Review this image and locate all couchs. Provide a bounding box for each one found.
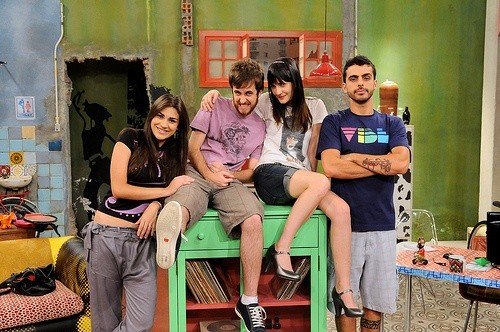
[0,236,93,332]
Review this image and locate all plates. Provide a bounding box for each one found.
[24,213,57,224]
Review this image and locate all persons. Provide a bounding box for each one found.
[79,93,195,332]
[152,56,267,332]
[200,56,366,319]
[314,55,410,332]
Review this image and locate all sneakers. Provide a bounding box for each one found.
[156,201,188,269]
[235,297,267,332]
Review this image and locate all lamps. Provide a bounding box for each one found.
[309,0,342,79]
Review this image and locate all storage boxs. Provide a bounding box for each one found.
[468,227,487,252]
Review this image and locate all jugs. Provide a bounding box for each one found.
[380,81,398,118]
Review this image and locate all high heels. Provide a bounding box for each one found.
[332,286,364,317]
[263,243,300,281]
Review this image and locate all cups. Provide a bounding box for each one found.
[449,254,465,273]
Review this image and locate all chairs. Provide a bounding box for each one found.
[459,221,500,332]
[397,209,438,314]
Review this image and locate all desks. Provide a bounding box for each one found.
[396,245,500,332]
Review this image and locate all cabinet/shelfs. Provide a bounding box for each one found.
[168,204,329,332]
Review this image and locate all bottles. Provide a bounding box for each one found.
[402,107,410,124]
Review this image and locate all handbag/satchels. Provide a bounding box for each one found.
[11,264,56,296]
